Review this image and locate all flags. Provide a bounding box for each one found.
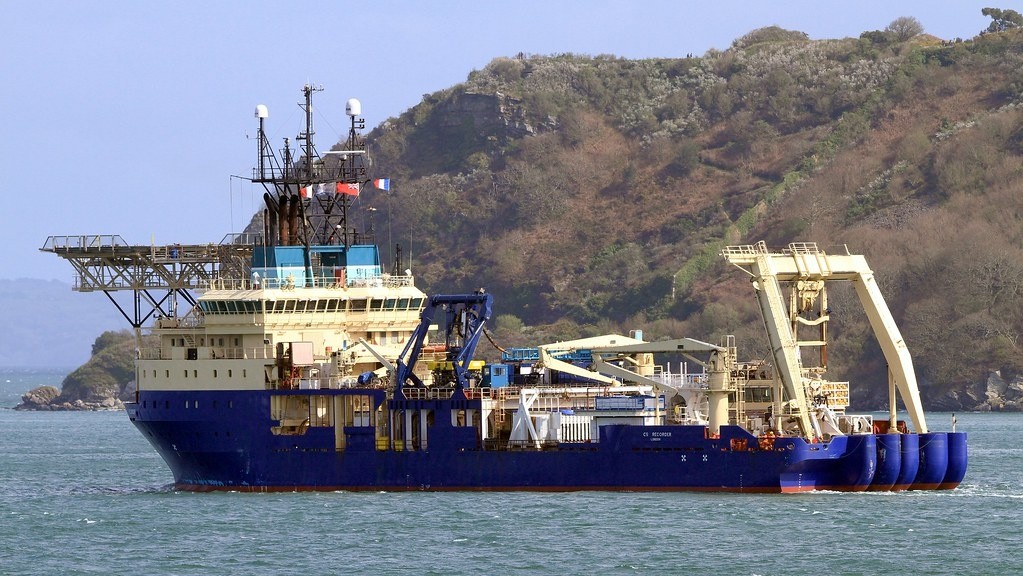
[372,177,392,192]
[336,181,360,198]
[314,181,336,200]
[299,184,317,200]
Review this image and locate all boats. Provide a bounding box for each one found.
[39,84,967,492]
